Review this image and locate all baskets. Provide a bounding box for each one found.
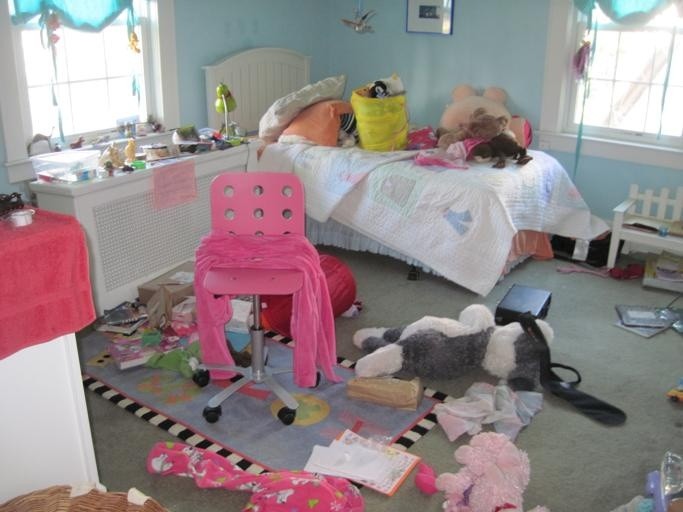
[1,485,169,512]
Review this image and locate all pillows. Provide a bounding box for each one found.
[276,98,353,147]
[256,68,347,141]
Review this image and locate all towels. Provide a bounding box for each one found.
[186,225,345,390]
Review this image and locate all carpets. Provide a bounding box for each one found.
[70,302,448,501]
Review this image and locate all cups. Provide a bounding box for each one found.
[10,208,35,227]
[658,226,670,236]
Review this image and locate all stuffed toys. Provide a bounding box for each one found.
[352,303,556,391]
[414,430,550,511]
[336,71,533,168]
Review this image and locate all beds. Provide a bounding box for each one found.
[197,40,613,297]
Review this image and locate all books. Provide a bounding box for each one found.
[613,248,683,338]
[101,322,186,371]
[92,300,149,336]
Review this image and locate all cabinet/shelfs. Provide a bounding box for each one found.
[0,205,102,508]
[24,130,251,316]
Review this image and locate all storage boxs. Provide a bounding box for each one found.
[133,259,197,309]
[217,298,253,353]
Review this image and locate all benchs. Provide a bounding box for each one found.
[600,180,681,277]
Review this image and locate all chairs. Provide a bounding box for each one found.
[187,170,321,424]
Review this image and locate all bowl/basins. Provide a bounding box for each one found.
[660,451,683,494]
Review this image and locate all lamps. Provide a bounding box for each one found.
[210,76,240,143]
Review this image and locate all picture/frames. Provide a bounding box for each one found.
[399,0,458,39]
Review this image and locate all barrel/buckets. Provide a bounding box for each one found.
[350,89,409,150]
